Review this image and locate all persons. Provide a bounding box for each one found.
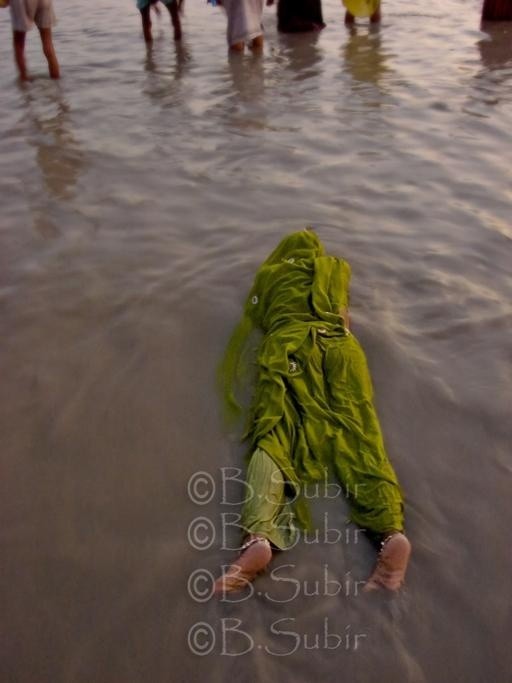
[223,57,268,131]
[341,0,381,26]
[207,0,264,55]
[474,27,512,74]
[142,50,188,110]
[135,0,187,50]
[19,81,84,200]
[0,0,60,81]
[346,25,384,85]
[211,228,411,595]
[481,0,512,26]
[266,0,327,34]
